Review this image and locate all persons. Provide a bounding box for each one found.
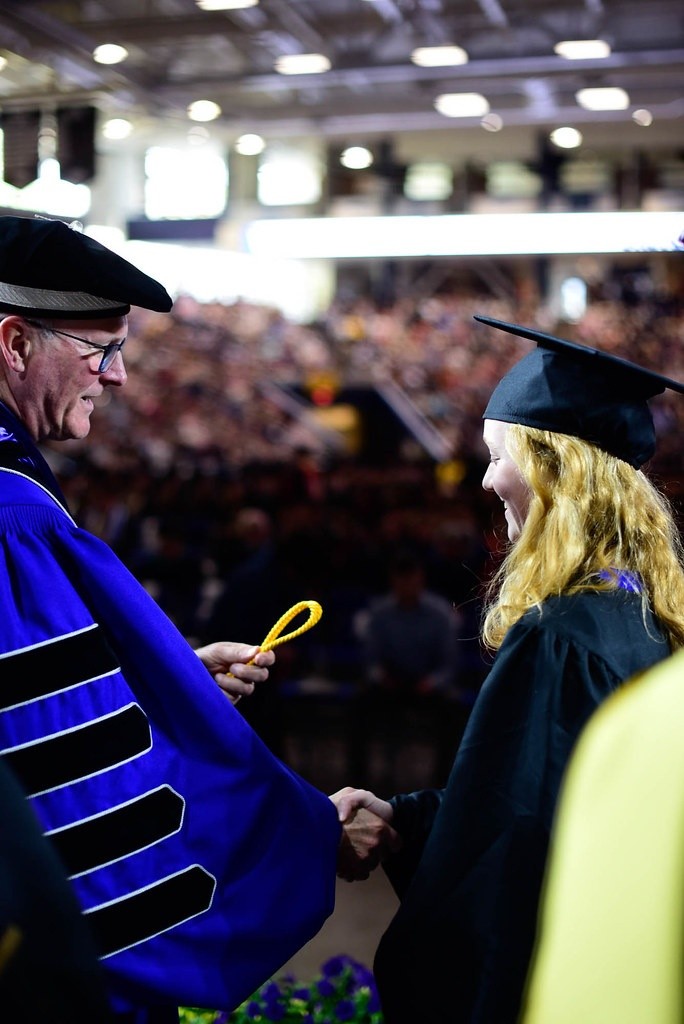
[337,350,683,1024]
[44,290,683,802]
[0,248,392,1024]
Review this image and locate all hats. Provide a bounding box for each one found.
[471,316,683,471]
[1,208,173,321]
